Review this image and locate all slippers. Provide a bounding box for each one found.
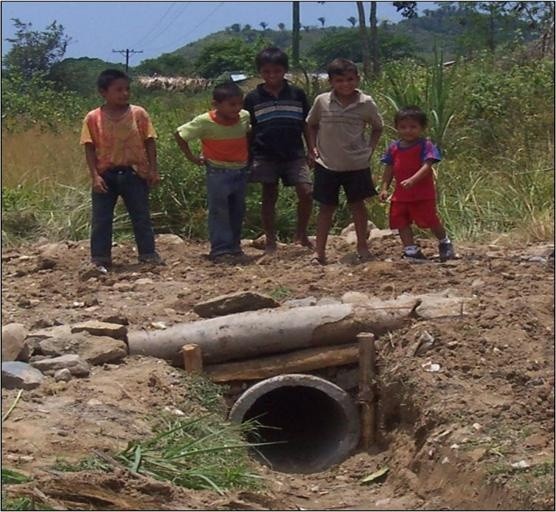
[311,254,326,266]
[296,241,314,249]
[357,251,374,262]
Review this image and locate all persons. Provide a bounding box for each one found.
[243,47,316,254]
[380,104,458,262]
[304,56,383,264]
[78,66,166,274]
[169,80,254,263]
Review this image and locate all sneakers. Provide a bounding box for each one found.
[231,253,248,265]
[137,251,164,264]
[214,253,232,264]
[438,238,454,262]
[405,244,422,258]
[91,255,111,268]
